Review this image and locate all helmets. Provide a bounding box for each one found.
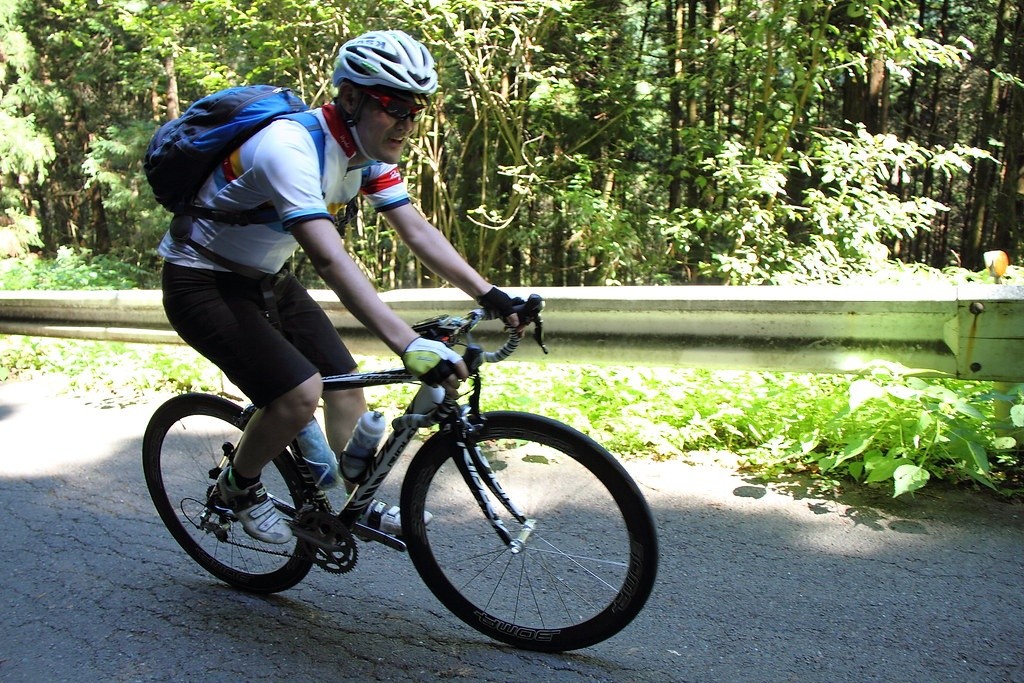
[333,29,438,110]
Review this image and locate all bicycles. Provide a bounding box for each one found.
[141,292,660,651]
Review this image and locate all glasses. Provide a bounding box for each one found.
[364,91,430,125]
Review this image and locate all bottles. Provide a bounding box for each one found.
[339,410,386,482]
[295,413,339,490]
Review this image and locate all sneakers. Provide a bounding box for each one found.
[353,498,433,542]
[218,467,294,546]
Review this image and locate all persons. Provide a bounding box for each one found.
[154,30,536,546]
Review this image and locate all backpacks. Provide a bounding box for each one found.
[145,83,323,231]
